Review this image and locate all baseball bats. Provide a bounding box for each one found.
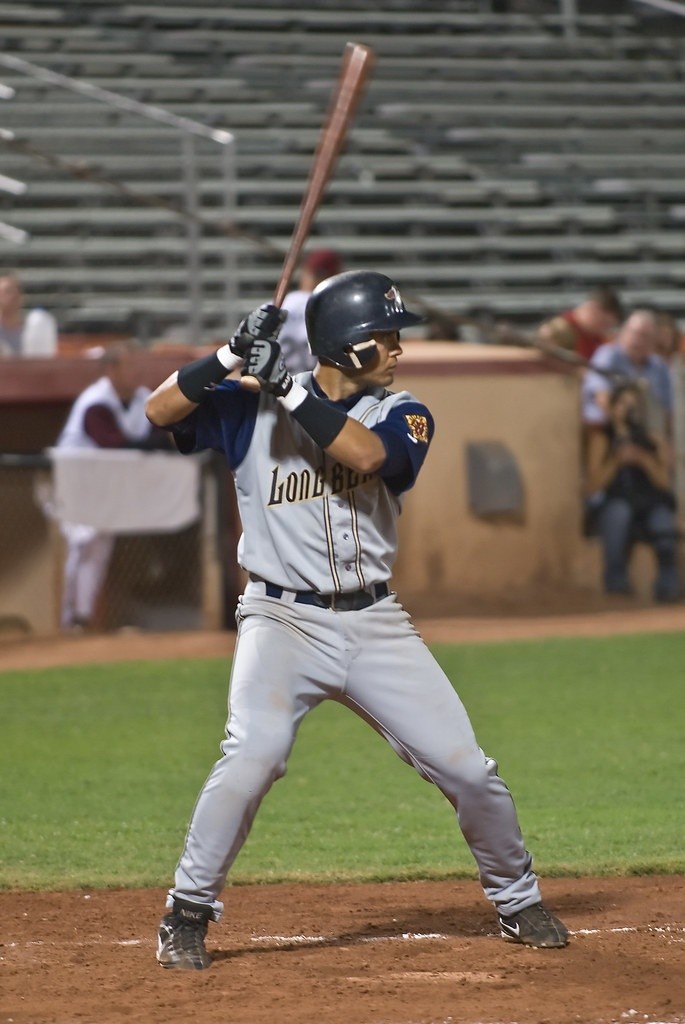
[239,40,380,392]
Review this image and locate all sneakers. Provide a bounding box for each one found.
[496,902,571,948]
[157,898,214,970]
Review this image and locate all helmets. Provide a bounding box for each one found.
[304,270,425,370]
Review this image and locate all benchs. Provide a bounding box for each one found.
[0,0,685,341]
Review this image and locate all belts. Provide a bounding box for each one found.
[263,580,389,612]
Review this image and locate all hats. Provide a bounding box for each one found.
[307,249,338,275]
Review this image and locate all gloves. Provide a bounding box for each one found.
[240,339,294,398]
[228,304,289,352]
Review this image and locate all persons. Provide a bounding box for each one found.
[52,336,177,637]
[144,269,572,969]
[0,271,66,361]
[261,250,348,380]
[536,283,684,607]
